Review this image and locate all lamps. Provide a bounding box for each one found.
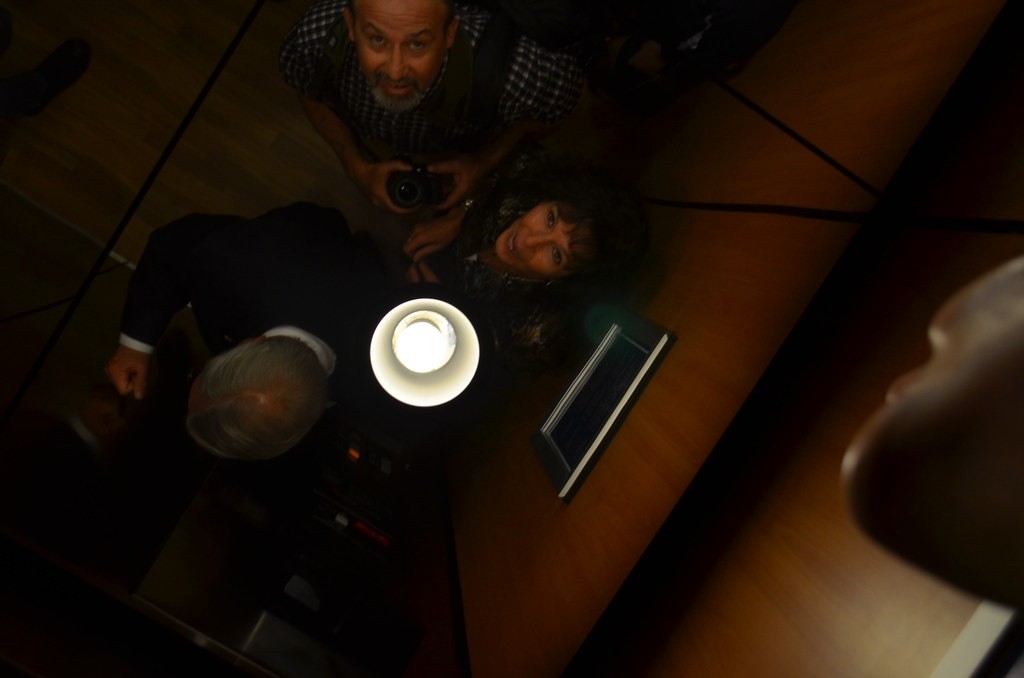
[369,297,480,409]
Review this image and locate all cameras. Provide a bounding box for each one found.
[387,163,443,209]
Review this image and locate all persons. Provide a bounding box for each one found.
[837,257,1024,617]
[106,0,794,489]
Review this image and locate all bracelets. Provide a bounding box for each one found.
[461,198,477,210]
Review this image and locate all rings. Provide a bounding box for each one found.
[408,236,412,241]
